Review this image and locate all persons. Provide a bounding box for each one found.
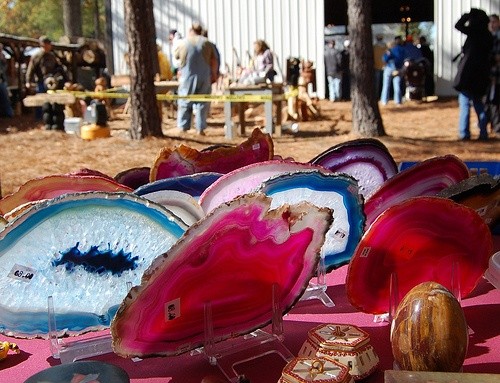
[174,25,218,136]
[487,14,500,134]
[242,40,318,119]
[451,8,495,141]
[323,32,434,106]
[0,36,117,134]
[156,29,182,122]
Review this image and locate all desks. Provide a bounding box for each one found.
[224,85,283,141]
[0,263,500,383]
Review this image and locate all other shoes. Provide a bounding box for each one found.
[458,137,470,141]
[479,135,488,140]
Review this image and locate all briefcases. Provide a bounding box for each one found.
[86,99,107,126]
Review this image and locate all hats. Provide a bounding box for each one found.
[39,36,51,43]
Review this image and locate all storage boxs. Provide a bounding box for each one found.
[277,324,378,382]
